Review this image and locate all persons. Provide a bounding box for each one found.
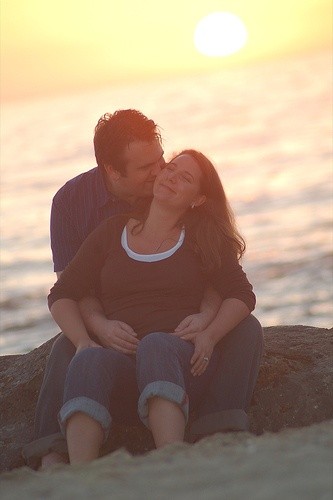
[19,109,264,474]
[46,148,258,467]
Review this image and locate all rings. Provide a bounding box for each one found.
[204,358,209,362]
